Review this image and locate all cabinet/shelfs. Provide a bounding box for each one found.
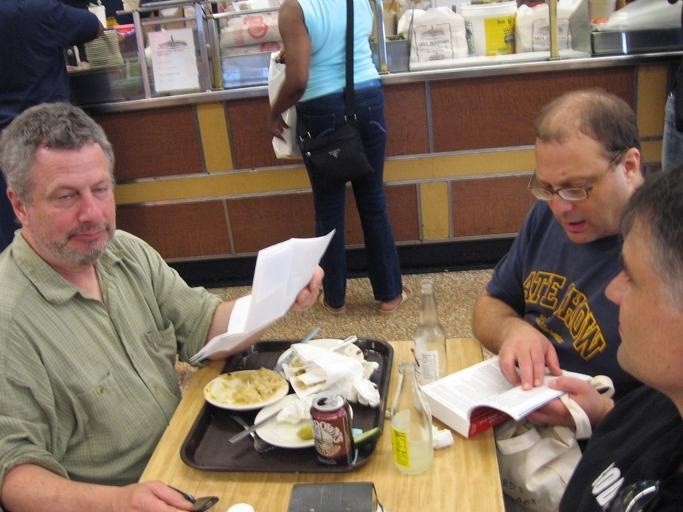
[68,0,682,290]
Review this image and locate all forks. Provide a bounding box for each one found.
[232,414,277,453]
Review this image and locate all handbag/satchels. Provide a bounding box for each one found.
[494,375,616,512]
[296,1,376,195]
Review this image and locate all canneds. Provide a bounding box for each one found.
[311,394,353,466]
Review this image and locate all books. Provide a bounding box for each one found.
[417,352,591,439]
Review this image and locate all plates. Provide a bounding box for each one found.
[275,338,365,397]
[254,393,353,450]
[204,369,289,411]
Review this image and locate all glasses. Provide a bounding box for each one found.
[527,153,622,202]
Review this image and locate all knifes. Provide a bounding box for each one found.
[272,325,321,373]
[228,408,281,445]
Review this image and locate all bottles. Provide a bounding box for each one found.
[413,279,447,412]
[392,363,433,476]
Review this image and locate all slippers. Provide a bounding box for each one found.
[376,285,412,315]
[317,290,347,316]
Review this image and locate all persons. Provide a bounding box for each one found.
[270,0,410,314]
[0,101,323,508]
[1,1,103,249]
[471,90,644,425]
[555,167,683,508]
